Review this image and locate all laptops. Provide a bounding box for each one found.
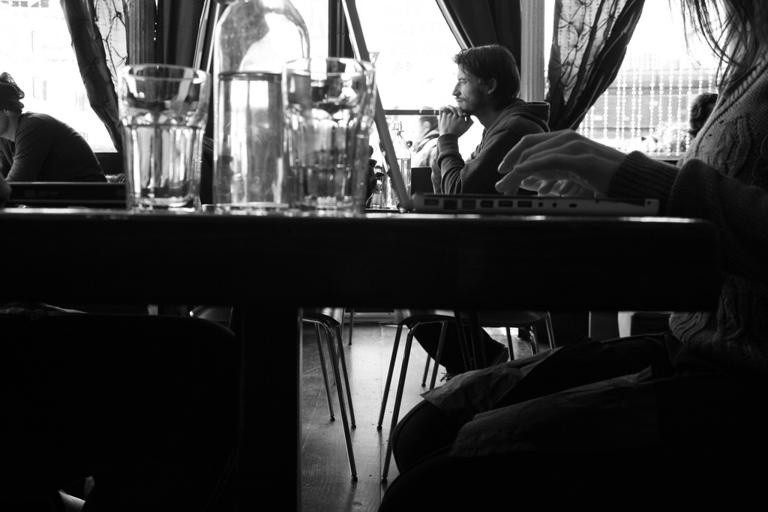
[342,0,659,216]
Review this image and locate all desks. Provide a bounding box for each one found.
[0,206,726,509]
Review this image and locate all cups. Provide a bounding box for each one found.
[116,63,212,212]
[286,54,375,212]
[370,138,415,210]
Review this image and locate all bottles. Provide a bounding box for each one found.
[216,1,306,213]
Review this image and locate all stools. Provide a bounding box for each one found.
[376,310,559,487]
[301,310,359,488]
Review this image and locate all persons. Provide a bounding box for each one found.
[398,45,550,378]
[416,107,440,190]
[381,1,767,511]
[368,145,376,199]
[687,92,716,140]
[0,70,106,203]
